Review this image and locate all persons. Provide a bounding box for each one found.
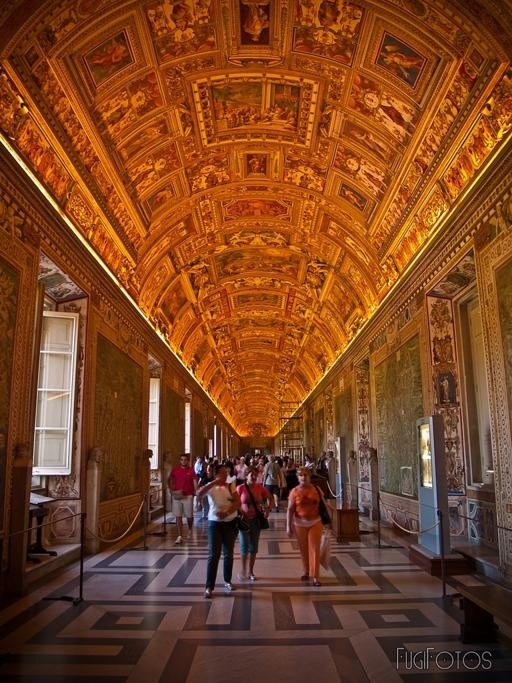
[167,450,339,599]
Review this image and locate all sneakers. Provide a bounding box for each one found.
[175,529,192,544]
[204,589,211,598]
[301,573,320,586]
[225,583,233,590]
[240,576,256,581]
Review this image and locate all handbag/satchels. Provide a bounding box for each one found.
[314,485,331,524]
[319,528,331,570]
[174,491,184,500]
[260,515,269,529]
[237,510,250,530]
